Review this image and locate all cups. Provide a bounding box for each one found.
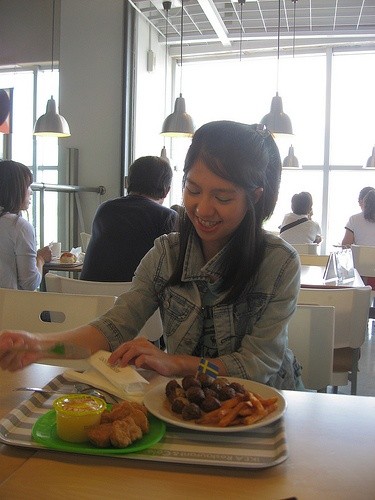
[49,242,61,258]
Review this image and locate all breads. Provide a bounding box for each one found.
[60,253,78,263]
[88,401,149,447]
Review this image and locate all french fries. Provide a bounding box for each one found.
[196,390,278,427]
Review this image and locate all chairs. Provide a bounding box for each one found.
[2,232,375,394]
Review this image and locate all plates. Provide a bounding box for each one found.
[31,409,167,454]
[142,376,287,432]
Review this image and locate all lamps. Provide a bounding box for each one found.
[282,0,301,169]
[32,1,71,137]
[257,0,294,138]
[159,0,196,137]
[363,145,375,169]
[159,1,173,169]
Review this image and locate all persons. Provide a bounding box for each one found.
[0,120,305,391]
[80,156,182,350]
[281,190,323,246]
[0,159,52,292]
[341,187,375,307]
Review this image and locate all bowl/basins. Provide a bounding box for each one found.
[53,394,106,443]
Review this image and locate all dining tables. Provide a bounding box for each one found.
[41,251,85,293]
[0,361,375,500]
[298,264,365,289]
